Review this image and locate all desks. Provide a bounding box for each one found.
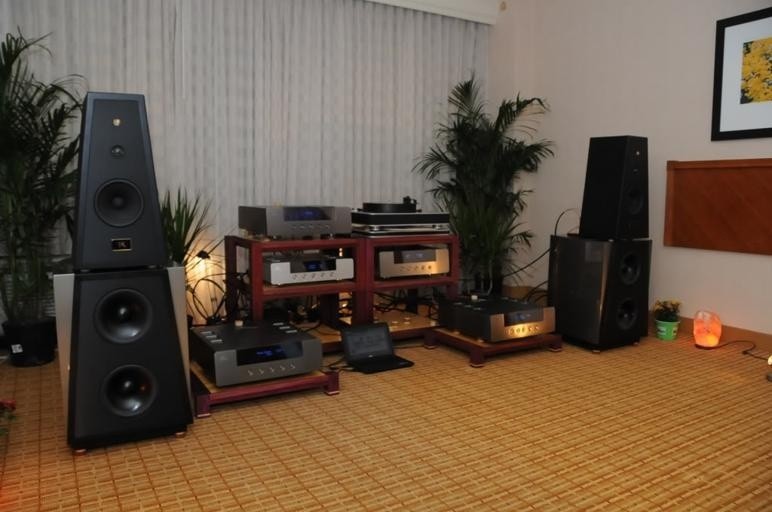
[217,228,462,356]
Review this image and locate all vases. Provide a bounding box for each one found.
[656,318,679,340]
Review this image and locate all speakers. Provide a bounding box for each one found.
[70,90,177,267]
[66,267,192,450]
[580,134,648,238]
[548,234,652,354]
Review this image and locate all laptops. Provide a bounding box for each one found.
[340,322,414,375]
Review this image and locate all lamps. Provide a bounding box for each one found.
[693,310,723,350]
[194,248,213,269]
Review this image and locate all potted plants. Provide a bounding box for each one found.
[159,187,235,329]
[412,72,555,297]
[0,25,83,366]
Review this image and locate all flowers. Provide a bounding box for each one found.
[653,298,681,321]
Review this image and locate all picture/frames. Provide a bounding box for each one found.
[711,7,772,142]
